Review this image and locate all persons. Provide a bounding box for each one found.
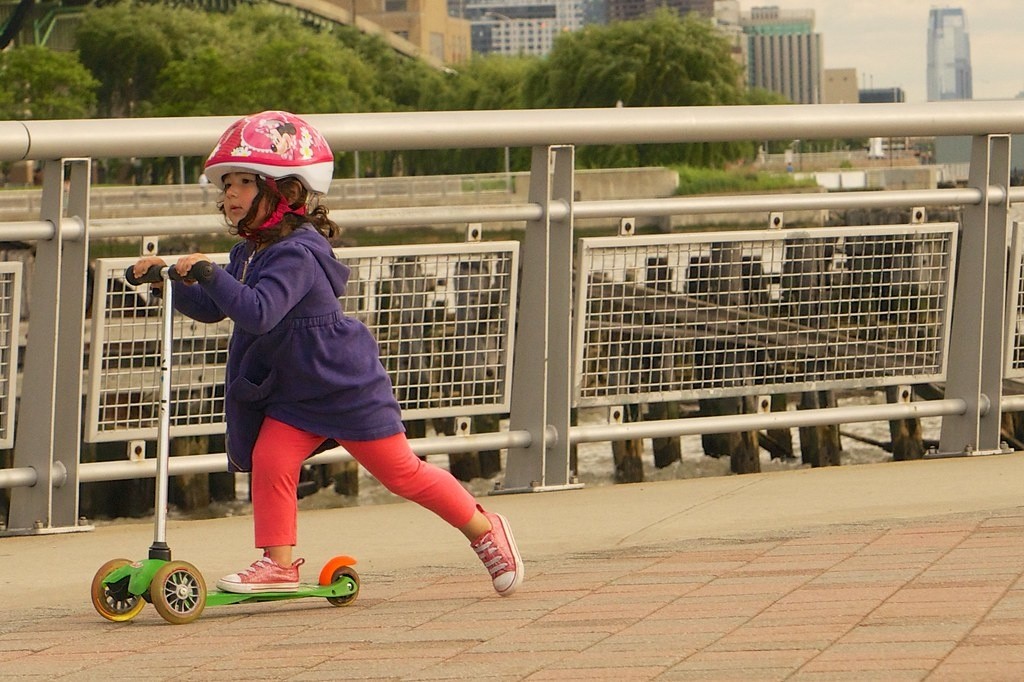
[133,111,525,596]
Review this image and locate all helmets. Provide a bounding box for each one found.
[204,111,334,195]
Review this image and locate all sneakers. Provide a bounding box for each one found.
[470,504,524,597]
[216,550,305,595]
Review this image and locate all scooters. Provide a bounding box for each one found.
[89,260,362,626]
